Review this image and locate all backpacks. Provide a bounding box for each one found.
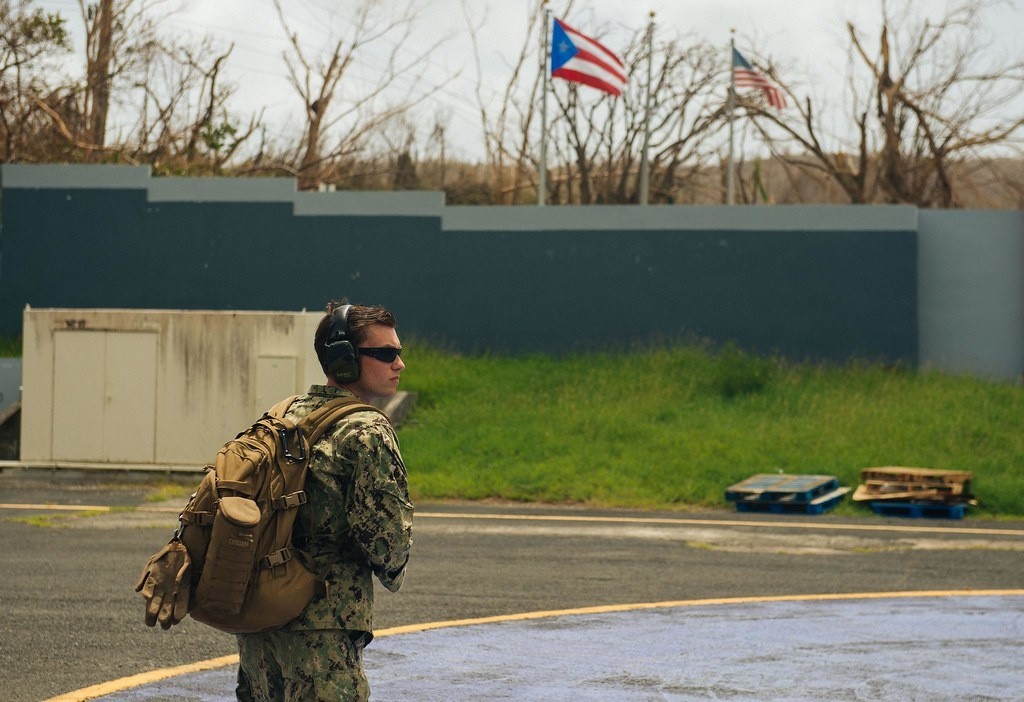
[178,412,321,637]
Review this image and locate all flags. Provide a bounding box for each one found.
[733,45,787,110]
[550,17,628,97]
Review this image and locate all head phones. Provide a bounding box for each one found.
[322,304,360,384]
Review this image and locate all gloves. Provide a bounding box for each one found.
[136,541,190,631]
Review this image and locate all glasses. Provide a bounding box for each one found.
[359,346,403,363]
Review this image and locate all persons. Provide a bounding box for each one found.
[234,303,415,702]
